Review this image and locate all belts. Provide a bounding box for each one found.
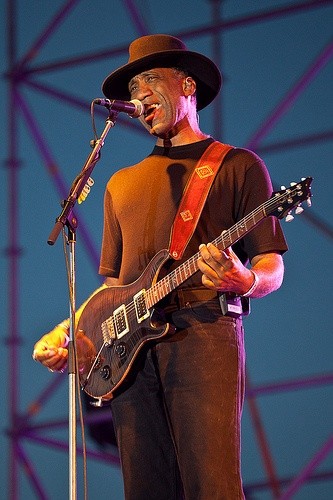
[156,287,239,311]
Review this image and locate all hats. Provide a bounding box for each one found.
[102,34,221,111]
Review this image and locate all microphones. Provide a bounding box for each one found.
[94,98,143,118]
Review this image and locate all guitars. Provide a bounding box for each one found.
[74,176,315,407]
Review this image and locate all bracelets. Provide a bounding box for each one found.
[241,271,259,298]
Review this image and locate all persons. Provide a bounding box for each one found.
[32,35,287,500]
